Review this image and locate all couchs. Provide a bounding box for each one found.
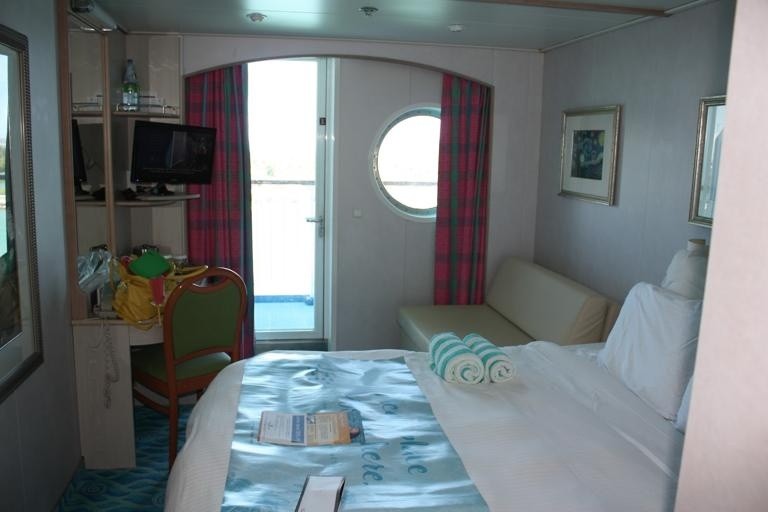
[398,257,620,352]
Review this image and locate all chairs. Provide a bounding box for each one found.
[130,267,248,474]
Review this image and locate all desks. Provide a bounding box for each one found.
[71,317,165,472]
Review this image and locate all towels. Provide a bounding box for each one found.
[427,332,484,386]
[463,334,514,383]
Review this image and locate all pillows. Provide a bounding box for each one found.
[598,281,704,423]
[676,377,694,433]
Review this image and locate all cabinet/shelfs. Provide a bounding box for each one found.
[109,25,199,318]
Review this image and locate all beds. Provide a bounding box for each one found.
[163,240,711,512]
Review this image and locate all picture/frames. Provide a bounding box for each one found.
[688,94,729,229]
[558,104,622,206]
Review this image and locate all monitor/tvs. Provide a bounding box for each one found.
[72,118,91,195]
[129,120,217,194]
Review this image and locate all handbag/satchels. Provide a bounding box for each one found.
[112,256,209,331]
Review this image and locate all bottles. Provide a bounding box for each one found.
[121,59,140,112]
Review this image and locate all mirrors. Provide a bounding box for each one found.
[0,25,43,404]
[58,5,116,321]
